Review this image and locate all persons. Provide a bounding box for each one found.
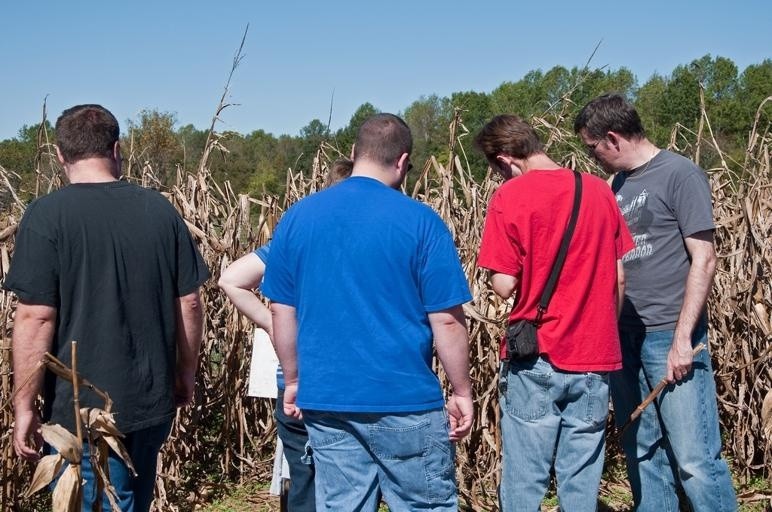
[0,104,212,510]
[265,112,476,512]
[476,113,635,512]
[573,91,740,512]
[216,160,352,512]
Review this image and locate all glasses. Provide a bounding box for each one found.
[587,137,606,160]
[407,159,414,172]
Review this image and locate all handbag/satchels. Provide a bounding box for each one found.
[506,319,539,367]
